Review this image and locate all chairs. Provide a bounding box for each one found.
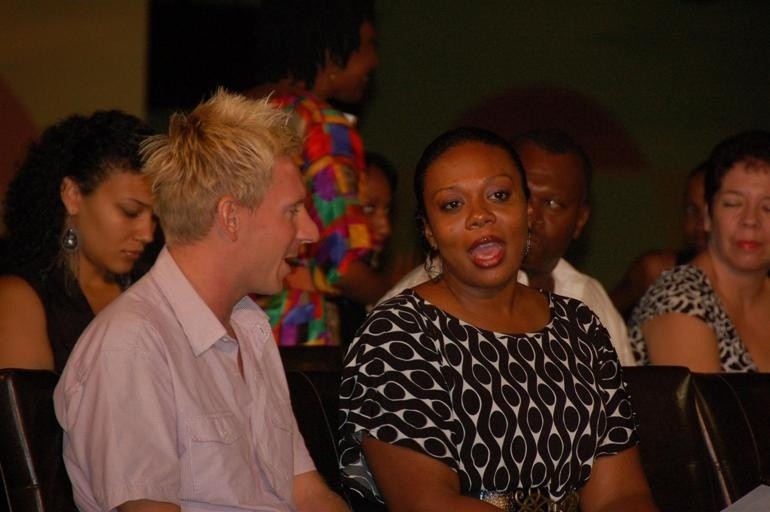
[2,344,769,510]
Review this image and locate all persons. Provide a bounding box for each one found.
[377,128,636,367]
[627,130,770,374]
[0,109,159,512]
[611,159,709,320]
[338,124,658,512]
[53,87,352,512]
[240,2,392,347]
[340,151,393,347]
